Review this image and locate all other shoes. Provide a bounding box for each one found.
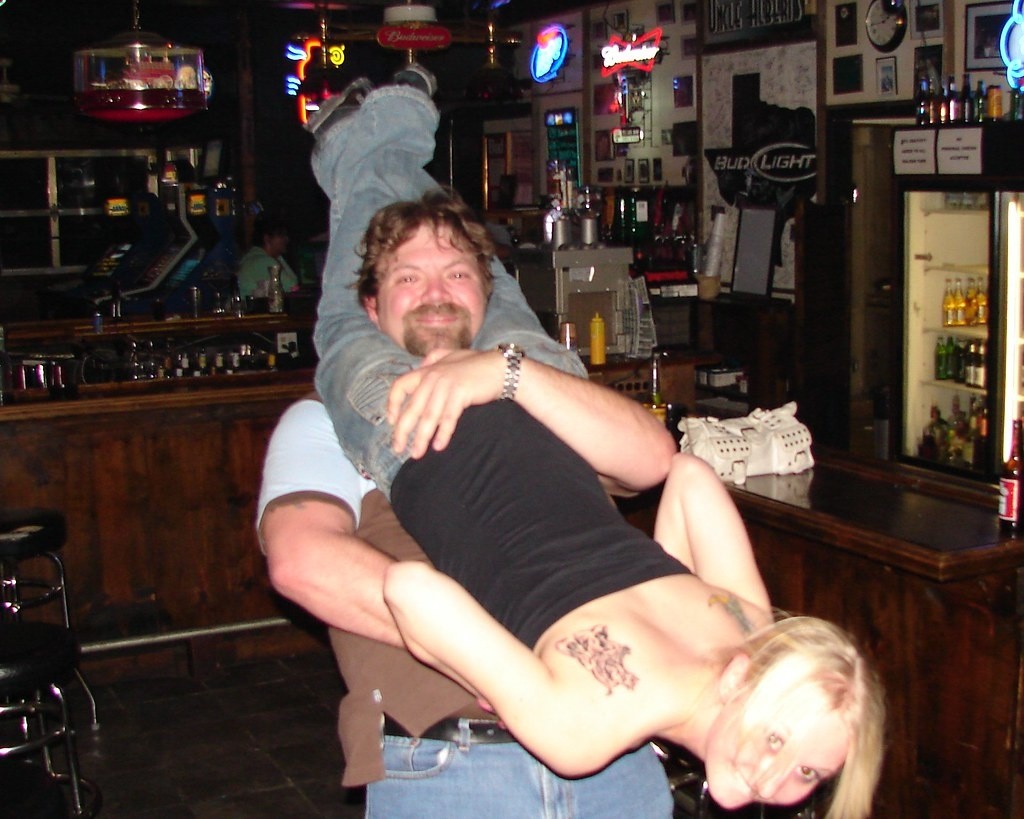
[303,78,373,140]
[395,63,438,99]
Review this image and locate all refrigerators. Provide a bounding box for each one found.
[890,126,1023,486]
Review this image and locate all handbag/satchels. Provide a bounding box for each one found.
[677,401,815,485]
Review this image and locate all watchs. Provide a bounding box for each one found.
[498,339,525,400]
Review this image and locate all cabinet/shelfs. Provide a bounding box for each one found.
[0,147,156,277]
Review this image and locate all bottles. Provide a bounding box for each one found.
[0,326,278,406]
[230,273,244,317]
[110,280,124,323]
[589,312,606,364]
[267,265,284,314]
[922,278,987,471]
[915,74,1024,125]
[999,421,1023,539]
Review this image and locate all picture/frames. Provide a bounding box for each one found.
[678,33,696,60]
[638,158,650,184]
[611,8,630,31]
[655,0,675,25]
[680,0,697,25]
[876,55,898,96]
[623,159,636,183]
[964,0,1014,73]
[595,130,616,161]
[909,0,944,39]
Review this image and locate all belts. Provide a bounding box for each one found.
[384,713,517,744]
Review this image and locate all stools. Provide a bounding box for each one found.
[0,620,102,819]
[0,507,71,628]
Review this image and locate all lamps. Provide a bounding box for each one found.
[73,0,209,122]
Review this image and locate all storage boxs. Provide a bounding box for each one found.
[694,368,744,388]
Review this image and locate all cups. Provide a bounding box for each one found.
[559,321,577,358]
[188,284,202,319]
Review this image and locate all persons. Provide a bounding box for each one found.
[237,202,303,314]
[257,187,678,819]
[307,63,884,819]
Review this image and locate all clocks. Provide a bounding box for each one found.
[866,0,908,52]
